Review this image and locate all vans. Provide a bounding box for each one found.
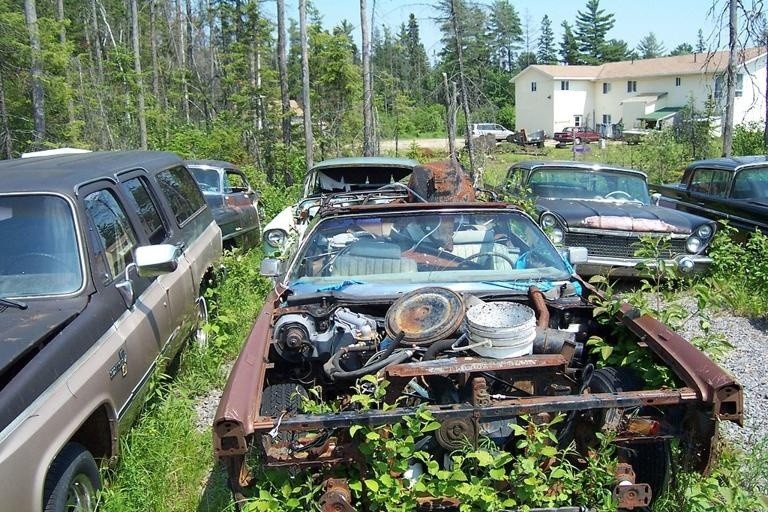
[465,123,515,142]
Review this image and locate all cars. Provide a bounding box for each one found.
[258,155,435,290]
[644,154,768,242]
[212,202,744,512]
[0,151,223,512]
[496,159,718,287]
[554,126,601,145]
[186,161,265,258]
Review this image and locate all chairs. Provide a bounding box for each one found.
[328,242,418,277]
[436,230,517,269]
